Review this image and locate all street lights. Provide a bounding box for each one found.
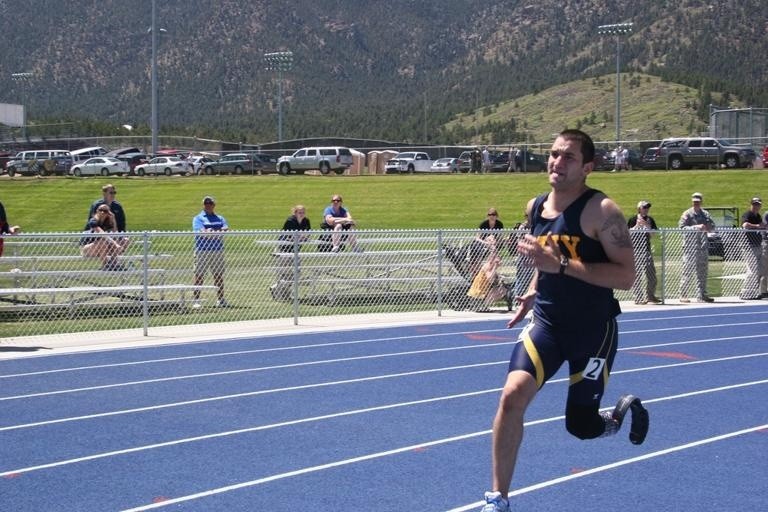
[597,22,634,143]
[264,51,295,139]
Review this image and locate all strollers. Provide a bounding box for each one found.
[444,240,519,313]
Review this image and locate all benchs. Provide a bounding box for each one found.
[0,238,223,322]
[254,236,518,313]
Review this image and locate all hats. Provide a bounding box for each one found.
[692,192,703,202]
[637,201,651,210]
[203,196,214,205]
[752,197,762,205]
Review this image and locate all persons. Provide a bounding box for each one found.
[464,143,526,175]
[324,196,368,253]
[608,144,635,173]
[629,191,767,304]
[461,209,533,311]
[85,183,131,253]
[270,203,311,303]
[481,131,635,512]
[190,196,232,308]
[0,200,22,256]
[80,205,127,270]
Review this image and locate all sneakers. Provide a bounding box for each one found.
[193,298,202,308]
[216,299,231,307]
[481,490,511,512]
[352,246,365,252]
[332,246,341,252]
[646,296,663,305]
[678,292,690,302]
[269,283,279,298]
[697,294,714,302]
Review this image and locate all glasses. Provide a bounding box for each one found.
[331,199,341,202]
[488,213,498,216]
[98,209,109,214]
[104,191,117,195]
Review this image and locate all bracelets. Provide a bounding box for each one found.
[558,257,569,275]
